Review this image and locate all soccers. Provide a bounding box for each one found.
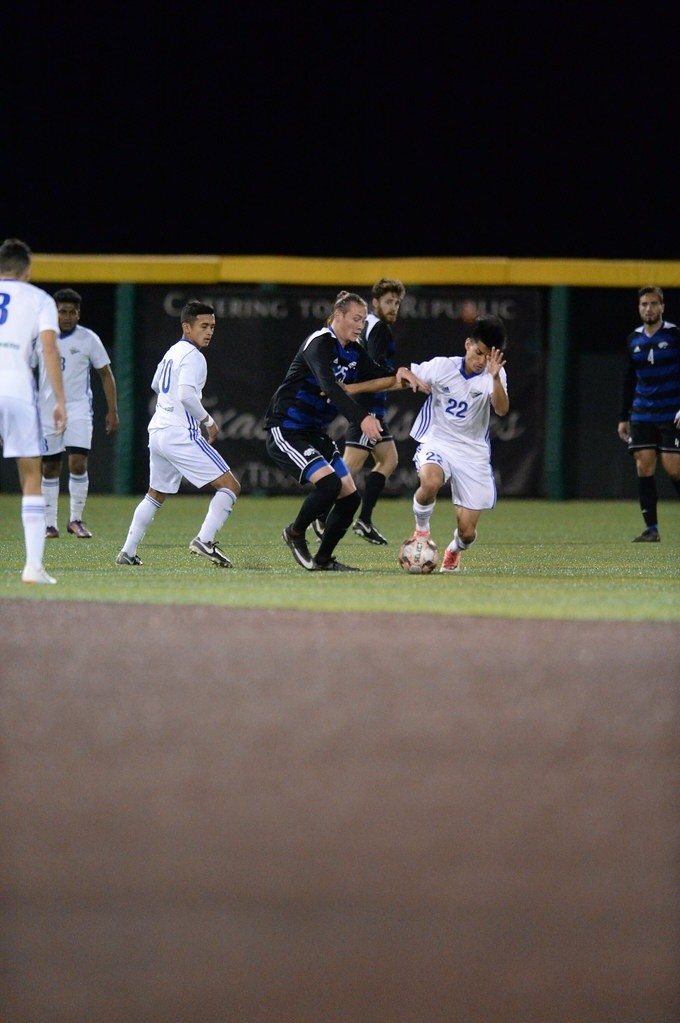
[397,535,440,575]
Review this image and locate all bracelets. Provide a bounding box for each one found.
[204,415,215,427]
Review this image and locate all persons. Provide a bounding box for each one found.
[28,288,120,537]
[113,299,241,568]
[618,286,680,544]
[320,314,510,573]
[262,290,433,572]
[312,278,405,545]
[0,239,67,585]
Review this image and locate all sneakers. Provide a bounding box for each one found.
[281,523,317,571]
[189,537,233,568]
[309,518,326,541]
[44,526,59,538]
[631,530,660,542]
[20,565,58,584]
[67,520,92,538]
[353,518,388,545]
[313,556,359,571]
[440,548,461,572]
[413,531,430,538]
[114,551,143,566]
